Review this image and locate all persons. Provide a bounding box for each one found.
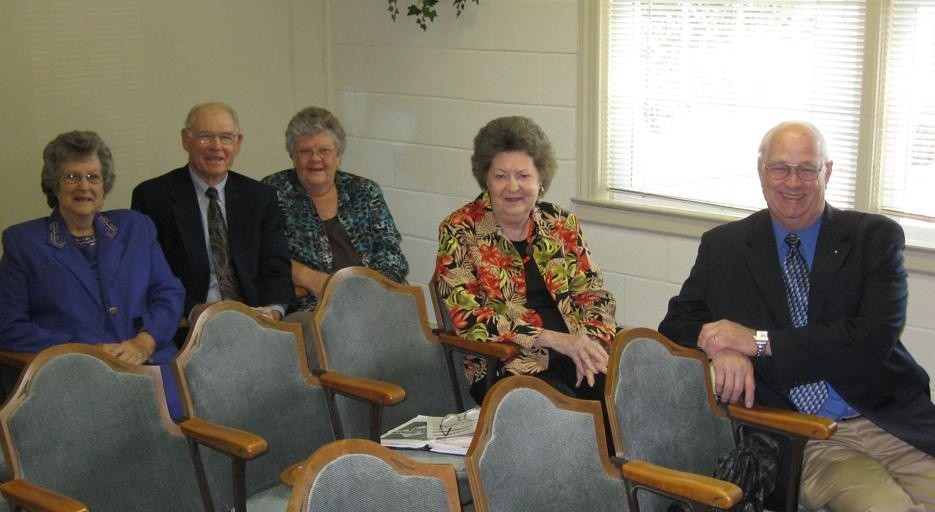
[657,122,935,512]
[132,102,296,321]
[0,130,187,423]
[433,116,624,457]
[259,108,409,313]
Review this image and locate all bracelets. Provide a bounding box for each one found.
[752,330,768,358]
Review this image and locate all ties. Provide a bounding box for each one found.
[783,235,829,416]
[205,188,246,302]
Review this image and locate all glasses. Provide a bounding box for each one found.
[191,132,236,146]
[760,159,824,182]
[63,170,103,186]
[439,408,480,437]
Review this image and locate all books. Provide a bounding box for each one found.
[380,406,482,456]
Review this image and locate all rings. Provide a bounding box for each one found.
[714,335,719,343]
